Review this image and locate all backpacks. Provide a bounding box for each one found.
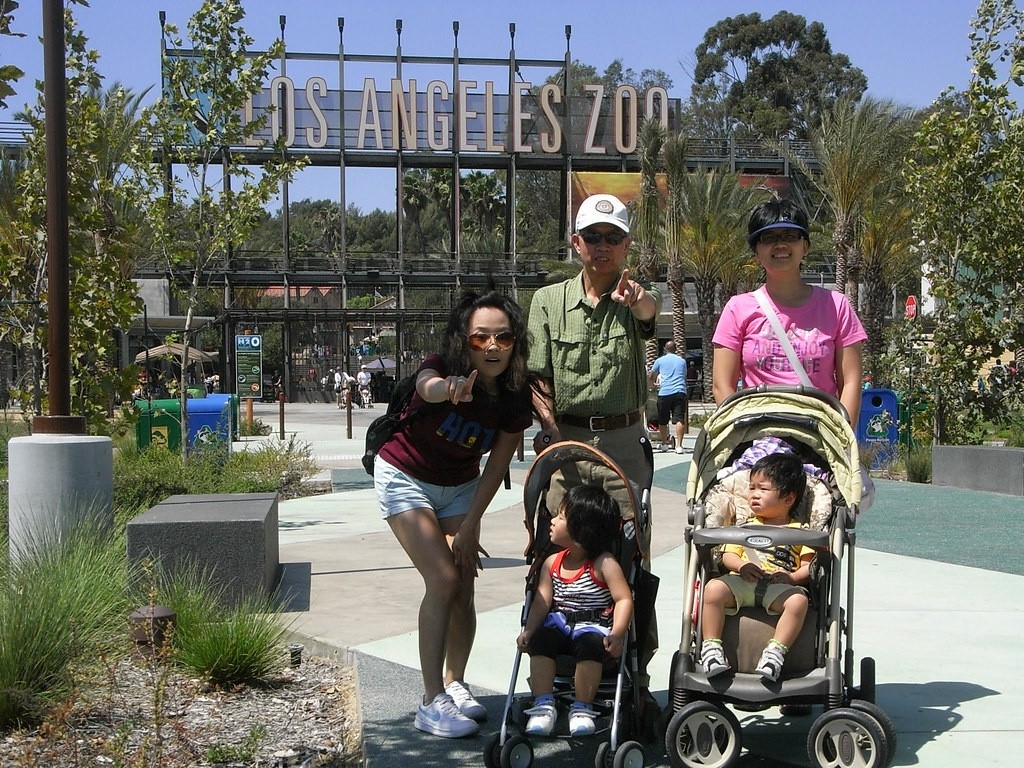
[360,355,463,478]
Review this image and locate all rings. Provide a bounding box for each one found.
[457,380,467,385]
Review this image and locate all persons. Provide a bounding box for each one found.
[864,377,873,390]
[373,291,535,738]
[649,341,688,455]
[516,485,633,735]
[687,362,698,401]
[712,199,867,718]
[140,368,169,396]
[978,359,1016,395]
[351,341,371,371]
[526,195,664,721]
[702,452,818,681]
[307,365,375,409]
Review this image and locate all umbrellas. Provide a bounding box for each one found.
[378,330,400,337]
[365,357,396,369]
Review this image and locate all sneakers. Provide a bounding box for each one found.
[567,706,601,737]
[675,446,684,454]
[657,443,669,451]
[413,693,481,739]
[523,704,558,737]
[700,642,732,680]
[443,679,488,722]
[754,647,785,683]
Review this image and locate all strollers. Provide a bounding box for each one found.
[482,434,666,768]
[337,384,355,410]
[659,381,900,768]
[356,384,374,410]
[643,383,676,452]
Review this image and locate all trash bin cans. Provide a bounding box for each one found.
[186,398,232,454]
[134,399,181,463]
[855,386,932,470]
[206,393,240,440]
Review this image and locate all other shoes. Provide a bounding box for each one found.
[337,405,340,409]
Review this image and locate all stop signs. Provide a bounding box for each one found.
[906,295,918,323]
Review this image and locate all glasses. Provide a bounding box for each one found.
[756,230,806,244]
[467,331,517,351]
[363,368,366,369]
[579,230,625,245]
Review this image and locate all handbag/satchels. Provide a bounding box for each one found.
[335,387,340,393]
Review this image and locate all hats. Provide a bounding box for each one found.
[747,222,810,246]
[361,364,367,368]
[575,193,630,238]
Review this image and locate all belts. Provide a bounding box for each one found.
[556,409,641,433]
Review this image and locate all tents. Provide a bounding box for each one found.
[136,343,216,398]
